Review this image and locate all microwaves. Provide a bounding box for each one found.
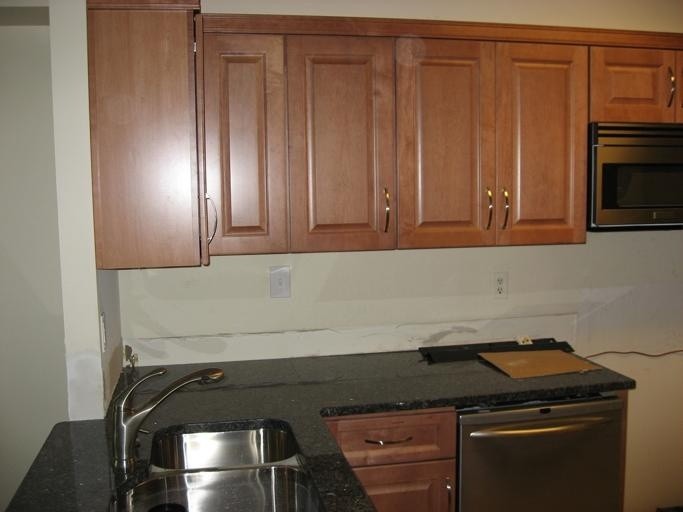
[588,122,683,229]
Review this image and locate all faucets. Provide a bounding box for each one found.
[110,367,225,472]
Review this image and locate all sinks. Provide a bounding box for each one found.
[111,465,326,511]
[148,417,300,472]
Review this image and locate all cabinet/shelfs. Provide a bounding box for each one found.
[86,0,218,270]
[195,33,290,256]
[332,407,456,510]
[396,37,588,251]
[287,33,397,252]
[590,45,682,124]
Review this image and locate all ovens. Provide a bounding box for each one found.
[459,398,624,512]
